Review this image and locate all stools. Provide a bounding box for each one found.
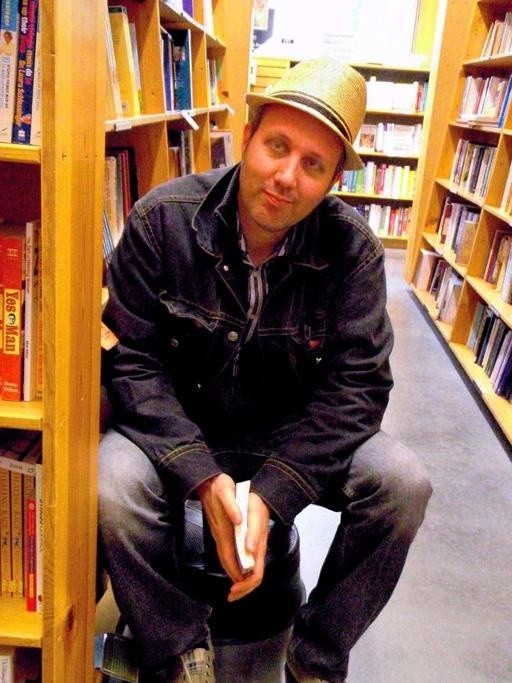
[166,497,305,682]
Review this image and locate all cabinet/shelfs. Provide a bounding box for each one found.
[0,0,257,683]
[251,55,432,249]
[403,1,512,447]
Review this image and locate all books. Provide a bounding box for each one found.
[436,194,479,263]
[105,146,138,260]
[366,77,428,112]
[448,138,497,199]
[348,204,410,234]
[357,122,422,154]
[161,0,192,17]
[105,4,142,118]
[159,24,193,111]
[332,162,416,196]
[167,129,195,178]
[1,222,39,403]
[414,248,463,324]
[482,229,512,301]
[1,3,43,147]
[210,128,234,168]
[1,647,41,683]
[456,76,511,126]
[500,154,512,216]
[0,430,44,615]
[476,9,510,61]
[207,57,219,106]
[464,303,512,401]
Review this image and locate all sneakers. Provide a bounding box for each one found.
[171,622,216,683]
[286,649,330,683]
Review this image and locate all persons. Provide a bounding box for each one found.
[97,55,432,683]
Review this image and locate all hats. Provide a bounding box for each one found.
[245,58,367,170]
